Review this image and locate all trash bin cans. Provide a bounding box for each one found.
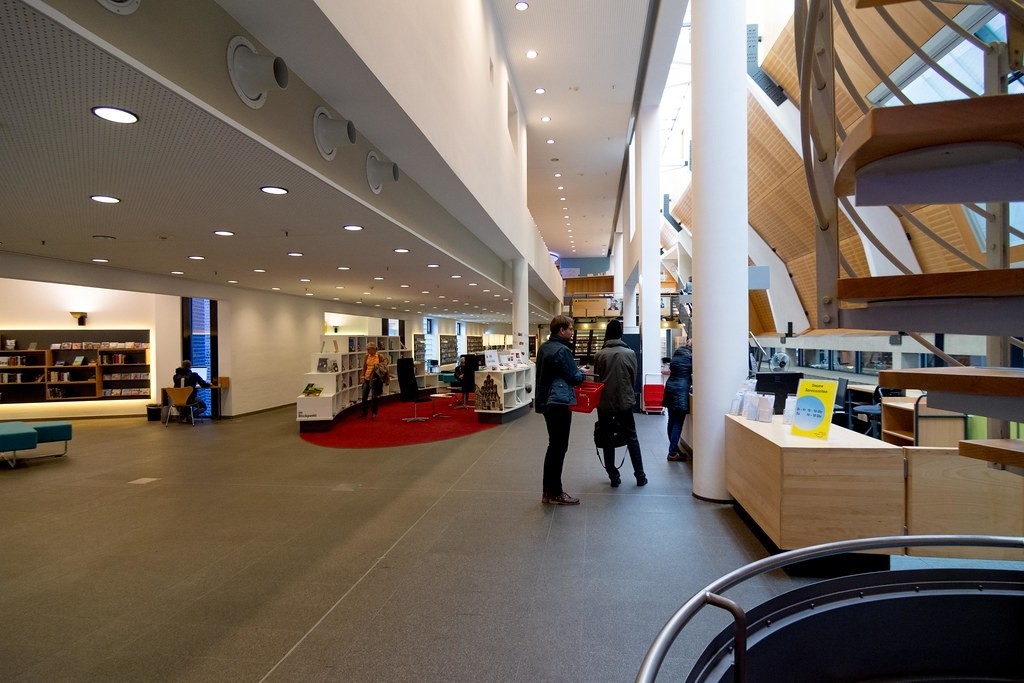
[146,404,160,421]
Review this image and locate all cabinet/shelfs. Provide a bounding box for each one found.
[724,387,1024,561]
[296,335,536,433]
[0,330,151,403]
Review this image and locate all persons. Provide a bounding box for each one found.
[593,319,648,487]
[534,315,591,505]
[686,277,692,308]
[173,360,213,423]
[359,342,387,420]
[608,303,618,310]
[454,357,465,402]
[662,338,692,462]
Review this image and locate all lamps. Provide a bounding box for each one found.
[549,251,560,261]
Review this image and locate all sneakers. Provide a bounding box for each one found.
[543,492,580,505]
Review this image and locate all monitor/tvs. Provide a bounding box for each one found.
[755,372,804,415]
[190,366,207,386]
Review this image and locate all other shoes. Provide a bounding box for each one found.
[668,451,688,462]
[178,415,196,424]
[610,478,621,487]
[636,478,647,487]
[357,414,368,419]
[372,414,376,419]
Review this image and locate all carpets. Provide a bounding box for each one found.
[300,392,502,449]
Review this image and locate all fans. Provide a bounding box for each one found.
[769,353,790,373]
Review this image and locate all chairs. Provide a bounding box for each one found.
[834,378,848,416]
[396,358,432,423]
[166,387,195,427]
[853,385,882,440]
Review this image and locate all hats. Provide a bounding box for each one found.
[605,320,623,340]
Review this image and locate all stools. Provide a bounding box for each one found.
[0,421,72,467]
[430,394,453,418]
[447,387,464,407]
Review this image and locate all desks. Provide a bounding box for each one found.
[161,386,222,421]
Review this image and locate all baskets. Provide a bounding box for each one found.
[568,373,605,413]
[643,384,665,411]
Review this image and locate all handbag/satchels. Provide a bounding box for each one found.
[594,418,627,448]
[373,376,383,396]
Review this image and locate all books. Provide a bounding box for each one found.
[0,339,150,399]
